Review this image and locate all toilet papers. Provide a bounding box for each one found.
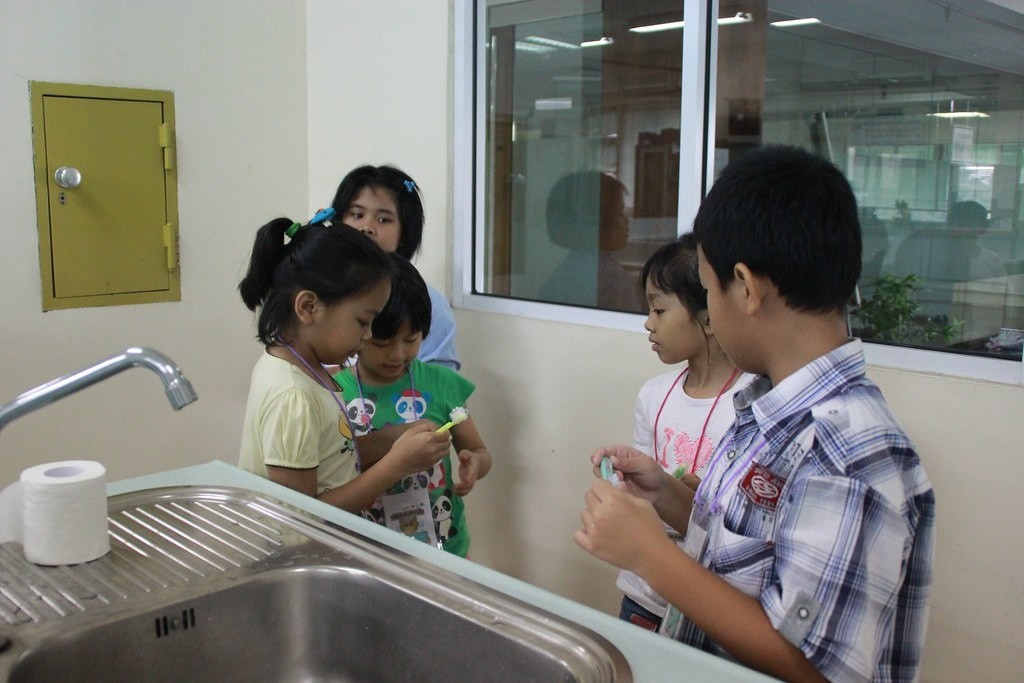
[0,460,111,565]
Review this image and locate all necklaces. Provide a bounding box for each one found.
[653,367,739,474]
[277,334,362,476]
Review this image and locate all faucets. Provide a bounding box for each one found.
[0,345,198,433]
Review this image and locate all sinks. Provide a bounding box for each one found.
[0,555,637,683]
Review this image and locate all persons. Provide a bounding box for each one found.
[237,218,451,515]
[576,148,934,682]
[330,254,494,562]
[614,234,756,635]
[536,169,646,313]
[320,165,461,377]
[855,201,1016,354]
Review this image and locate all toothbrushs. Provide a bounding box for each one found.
[435,406,468,432]
